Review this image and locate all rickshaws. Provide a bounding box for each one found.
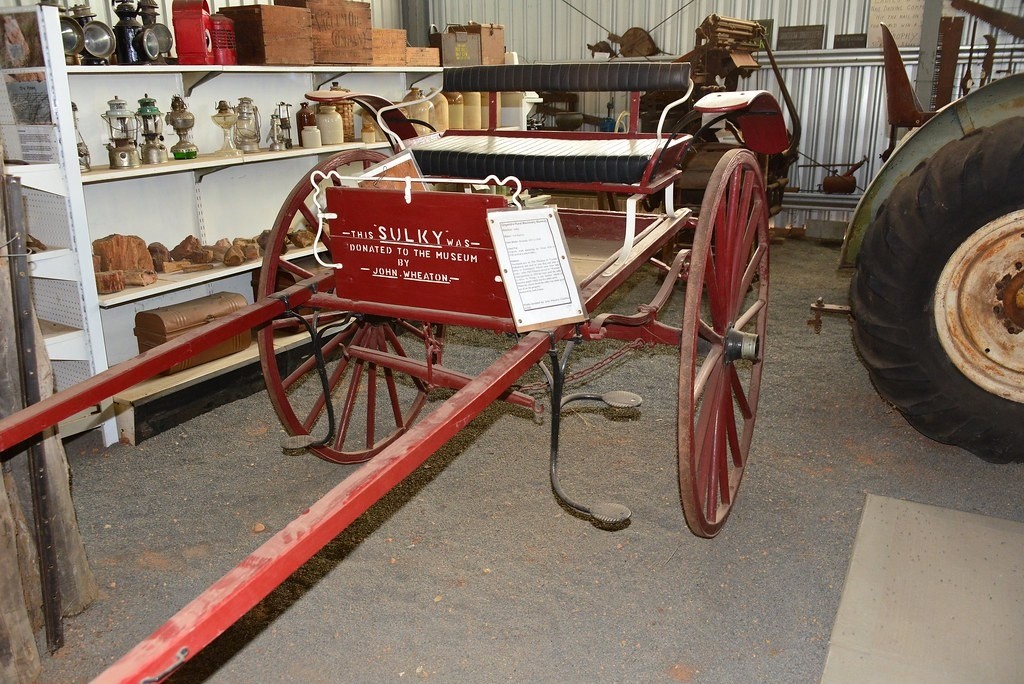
[0,59,796,684]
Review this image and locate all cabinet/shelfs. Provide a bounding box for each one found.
[65,6,546,447]
[0,5,120,448]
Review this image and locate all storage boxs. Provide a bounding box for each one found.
[428,21,505,67]
[273,0,372,65]
[133,291,251,376]
[219,4,314,65]
[250,254,346,332]
[406,48,439,67]
[372,28,408,66]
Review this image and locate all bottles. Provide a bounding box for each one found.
[296,103,316,147]
[420,96,435,132]
[461,92,481,129]
[426,88,449,132]
[301,126,322,149]
[481,92,502,129]
[402,88,429,136]
[316,106,344,144]
[440,89,463,130]
[210,14,238,65]
[361,123,375,143]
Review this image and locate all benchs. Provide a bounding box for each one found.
[376,64,695,276]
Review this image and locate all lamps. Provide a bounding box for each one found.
[210,97,262,155]
[102,95,199,169]
[40,0,173,66]
[265,102,294,152]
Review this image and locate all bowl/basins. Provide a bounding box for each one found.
[823,175,856,194]
[555,112,583,131]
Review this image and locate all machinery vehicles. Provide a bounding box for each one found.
[805,71,1024,464]
